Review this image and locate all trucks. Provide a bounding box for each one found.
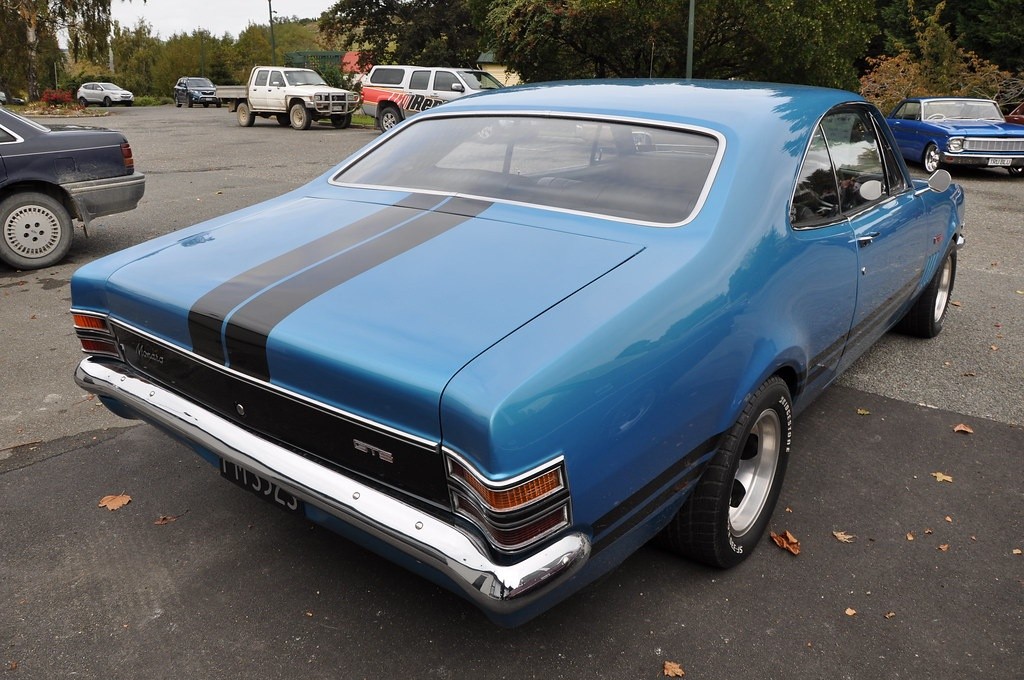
[214,65,361,130]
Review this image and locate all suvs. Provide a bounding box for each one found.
[173,76,222,108]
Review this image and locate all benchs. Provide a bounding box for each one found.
[578,158,713,193]
[389,157,689,222]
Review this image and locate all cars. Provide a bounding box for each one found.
[876,97,1024,179]
[0,108,147,271]
[77,81,135,108]
[979,101,1024,124]
[71,77,967,630]
[0,91,7,106]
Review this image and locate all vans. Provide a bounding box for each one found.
[363,64,507,134]
